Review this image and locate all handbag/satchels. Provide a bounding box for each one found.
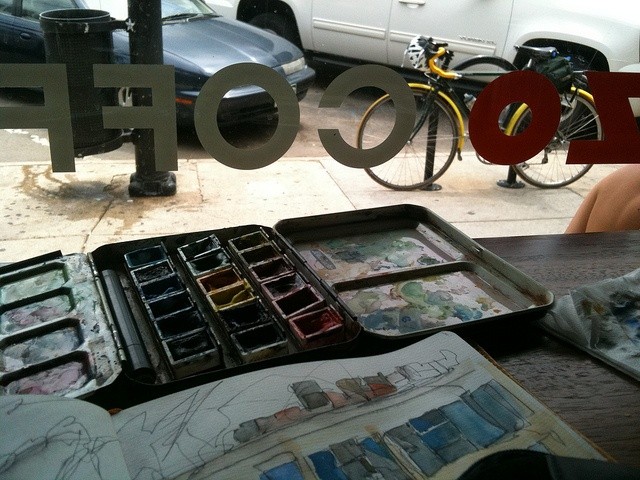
[534,57,575,95]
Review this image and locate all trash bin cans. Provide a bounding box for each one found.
[38,7,123,157]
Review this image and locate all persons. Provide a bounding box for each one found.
[563,163,640,234]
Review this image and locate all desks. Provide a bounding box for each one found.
[464,228,639,478]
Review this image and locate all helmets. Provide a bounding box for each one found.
[407,34,435,73]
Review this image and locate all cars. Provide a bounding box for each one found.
[203,0,640,96]
[1,0,318,140]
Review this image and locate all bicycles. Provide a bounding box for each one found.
[357,36,607,192]
